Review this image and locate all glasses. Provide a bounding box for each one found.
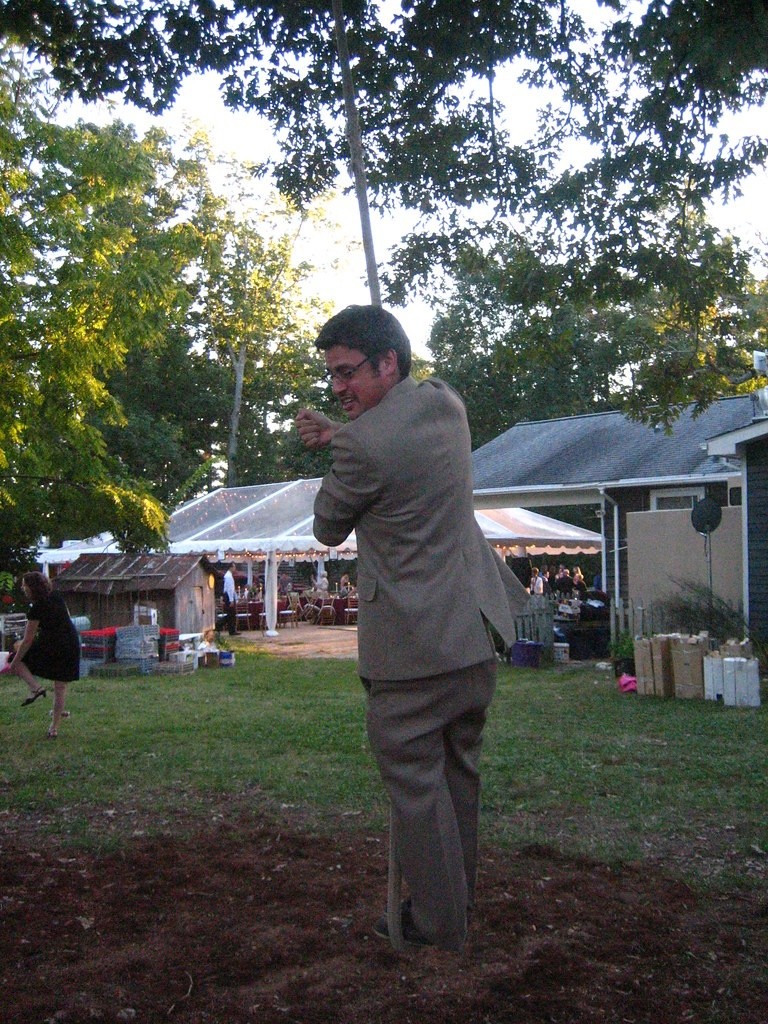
[321,357,370,386]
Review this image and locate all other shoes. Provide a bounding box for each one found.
[229,631,241,635]
[215,622,223,631]
[375,898,438,947]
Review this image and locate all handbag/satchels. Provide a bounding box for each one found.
[530,591,534,595]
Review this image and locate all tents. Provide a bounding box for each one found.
[35,476,607,566]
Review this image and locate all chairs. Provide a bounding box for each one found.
[215,596,228,632]
[286,592,308,625]
[344,596,359,625]
[258,601,268,631]
[278,594,298,628]
[234,598,251,630]
[300,590,337,626]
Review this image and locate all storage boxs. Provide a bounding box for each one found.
[509,638,544,669]
[553,643,569,663]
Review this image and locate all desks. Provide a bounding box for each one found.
[236,601,264,631]
[318,596,358,624]
[281,596,317,622]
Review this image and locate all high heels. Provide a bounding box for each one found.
[21,686,46,706]
[47,729,57,738]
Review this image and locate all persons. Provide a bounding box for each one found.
[215,561,242,636]
[340,571,355,598]
[311,570,329,595]
[253,573,294,595]
[242,588,250,599]
[293,303,534,951]
[593,567,610,592]
[529,564,589,605]
[6,571,83,741]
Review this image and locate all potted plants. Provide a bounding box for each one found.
[607,629,636,677]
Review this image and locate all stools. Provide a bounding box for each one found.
[169,649,198,674]
